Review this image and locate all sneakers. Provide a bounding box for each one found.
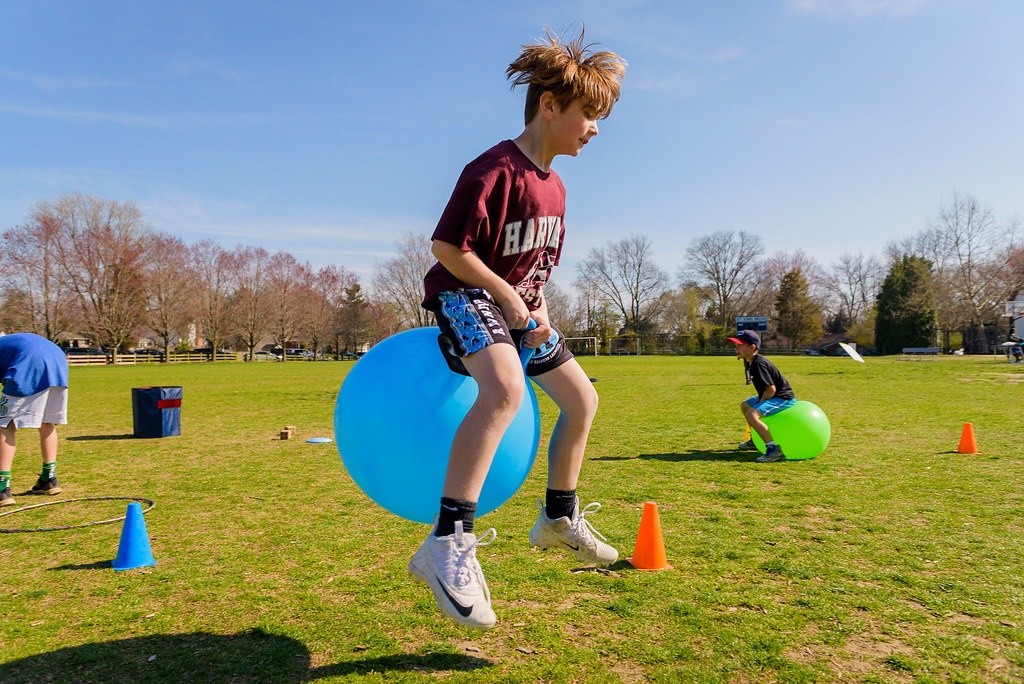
[32,474,62,495]
[0,487,16,507]
[739,438,758,450]
[408,514,497,629]
[529,494,619,564]
[757,444,786,463]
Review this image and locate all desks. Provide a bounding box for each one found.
[990,345,1024,364]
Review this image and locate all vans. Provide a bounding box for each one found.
[63,346,111,359]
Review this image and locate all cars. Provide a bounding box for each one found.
[135,348,163,356]
[244,352,276,360]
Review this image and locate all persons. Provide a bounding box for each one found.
[0,333,69,507]
[1008,328,1024,362]
[407,22,628,629]
[726,329,796,463]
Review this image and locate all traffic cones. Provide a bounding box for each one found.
[953,423,981,454]
[111,502,157,570]
[625,502,675,572]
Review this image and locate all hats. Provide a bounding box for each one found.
[726,330,761,349]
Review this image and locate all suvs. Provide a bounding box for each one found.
[286,348,320,360]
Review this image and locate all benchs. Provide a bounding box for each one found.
[615,351,631,356]
[903,348,939,355]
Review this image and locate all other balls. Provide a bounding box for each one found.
[333,326,543,527]
[751,400,831,461]
